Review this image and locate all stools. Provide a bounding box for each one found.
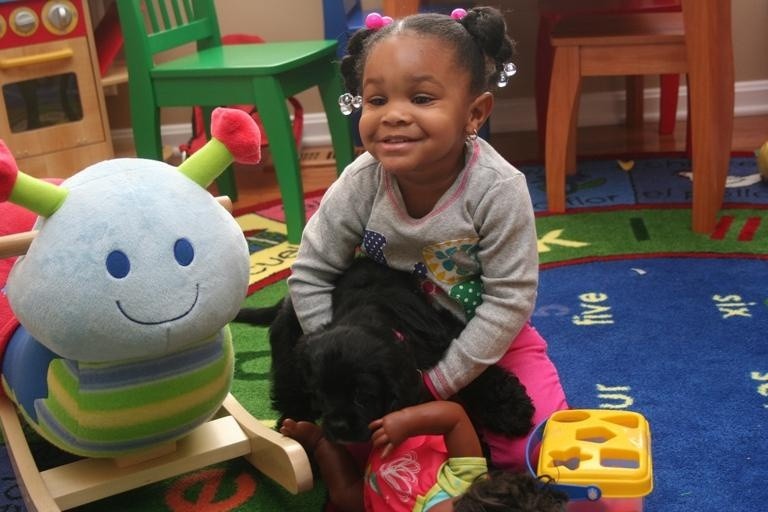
[541,0,685,137]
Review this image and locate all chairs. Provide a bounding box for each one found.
[116,1,360,254]
[535,1,735,235]
[319,0,497,151]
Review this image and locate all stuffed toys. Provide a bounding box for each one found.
[0,104,315,512]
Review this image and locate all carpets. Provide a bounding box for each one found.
[2,143,766,511]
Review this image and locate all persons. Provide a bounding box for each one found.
[276,401,569,511]
[291,5,582,472]
